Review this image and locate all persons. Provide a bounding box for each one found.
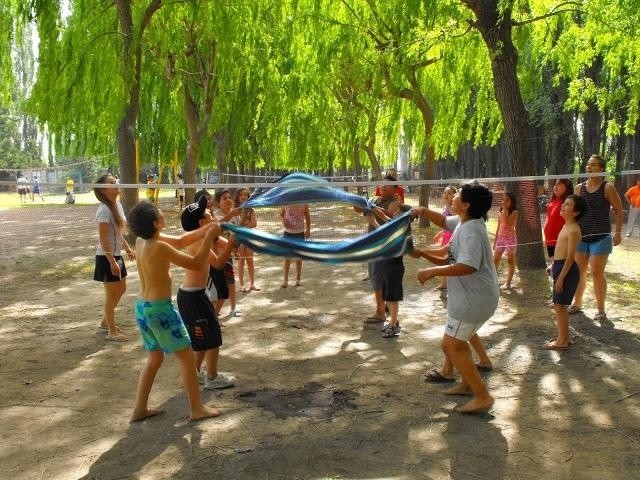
[493,192,519,288]
[543,178,574,263]
[354,169,413,338]
[145,174,160,203]
[180,187,258,390]
[202,178,207,191]
[17,174,45,204]
[411,179,500,414]
[567,154,624,321]
[540,194,588,351]
[126,198,222,422]
[175,174,185,205]
[279,203,311,289]
[66,177,74,194]
[65,191,76,204]
[92,174,136,343]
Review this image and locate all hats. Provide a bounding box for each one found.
[180,194,208,231]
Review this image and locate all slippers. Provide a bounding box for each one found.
[99,281,305,390]
[363,306,606,382]
